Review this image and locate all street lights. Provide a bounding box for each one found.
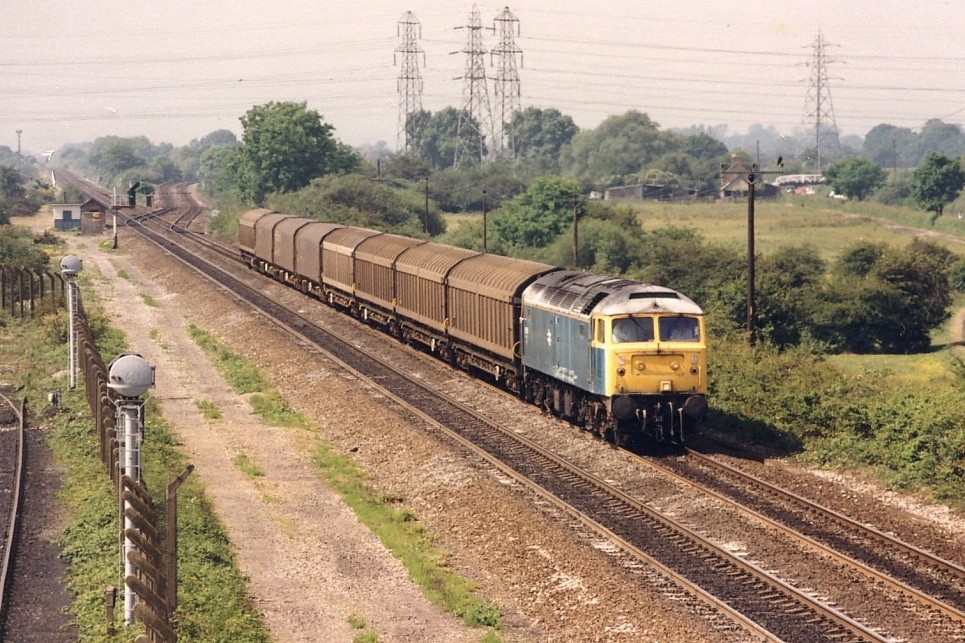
[721,156,786,345]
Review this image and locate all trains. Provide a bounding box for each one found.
[237,208,707,449]
